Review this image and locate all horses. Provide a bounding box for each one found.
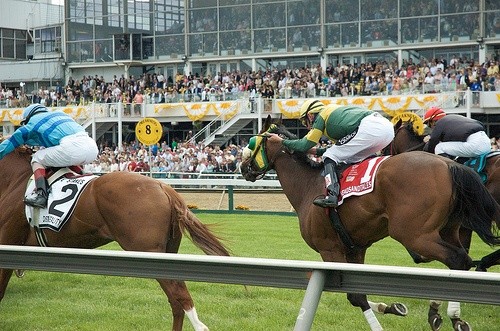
[240,113,500,331]
[379,116,500,272]
[1,145,249,331]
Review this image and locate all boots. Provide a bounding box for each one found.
[313,162,339,208]
[23,168,49,208]
[70,165,85,175]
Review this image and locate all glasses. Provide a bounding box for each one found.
[301,115,311,127]
[427,121,433,127]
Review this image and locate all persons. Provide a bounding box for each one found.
[82,131,251,178]
[267,98,395,209]
[488,133,500,151]
[65,35,158,64]
[159,0,500,56]
[26,145,45,152]
[0,54,500,117]
[423,108,491,158]
[0,104,99,208]
[306,136,334,164]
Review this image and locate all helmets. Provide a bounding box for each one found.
[22,103,48,125]
[299,98,324,120]
[423,108,446,123]
